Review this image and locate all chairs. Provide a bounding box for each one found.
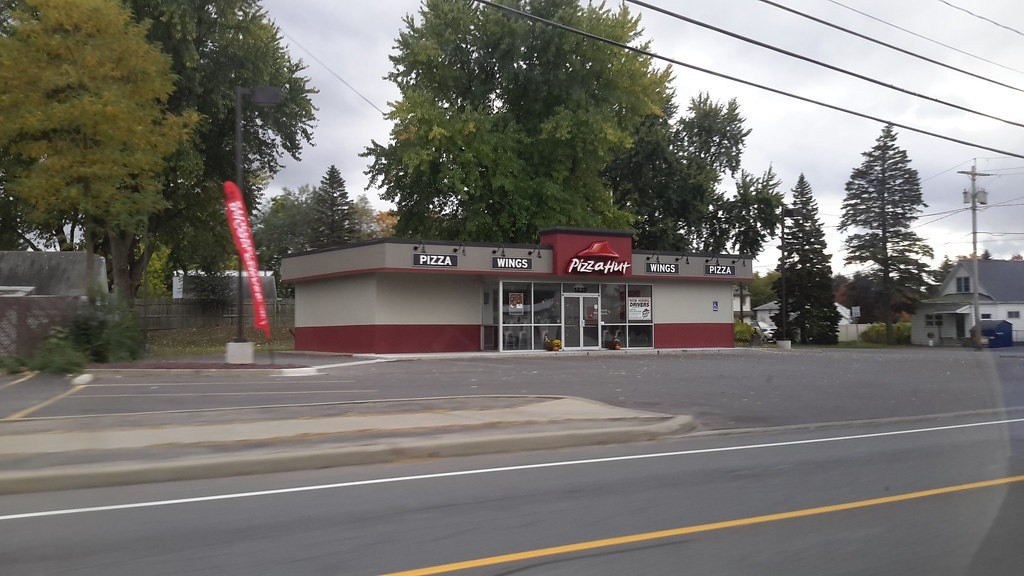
[515,319,530,350]
[503,318,514,350]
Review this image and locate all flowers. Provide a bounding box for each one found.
[551,339,561,346]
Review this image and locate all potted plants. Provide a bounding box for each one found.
[733,320,755,347]
[612,338,621,349]
[927,332,935,347]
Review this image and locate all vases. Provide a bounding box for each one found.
[552,345,560,350]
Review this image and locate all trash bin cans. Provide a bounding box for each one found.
[969,320,1013,348]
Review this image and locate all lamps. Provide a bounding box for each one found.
[646,251,659,264]
[413,239,426,253]
[731,255,745,267]
[528,246,542,258]
[492,243,505,257]
[453,242,466,256]
[705,255,719,266]
[675,252,689,264]
